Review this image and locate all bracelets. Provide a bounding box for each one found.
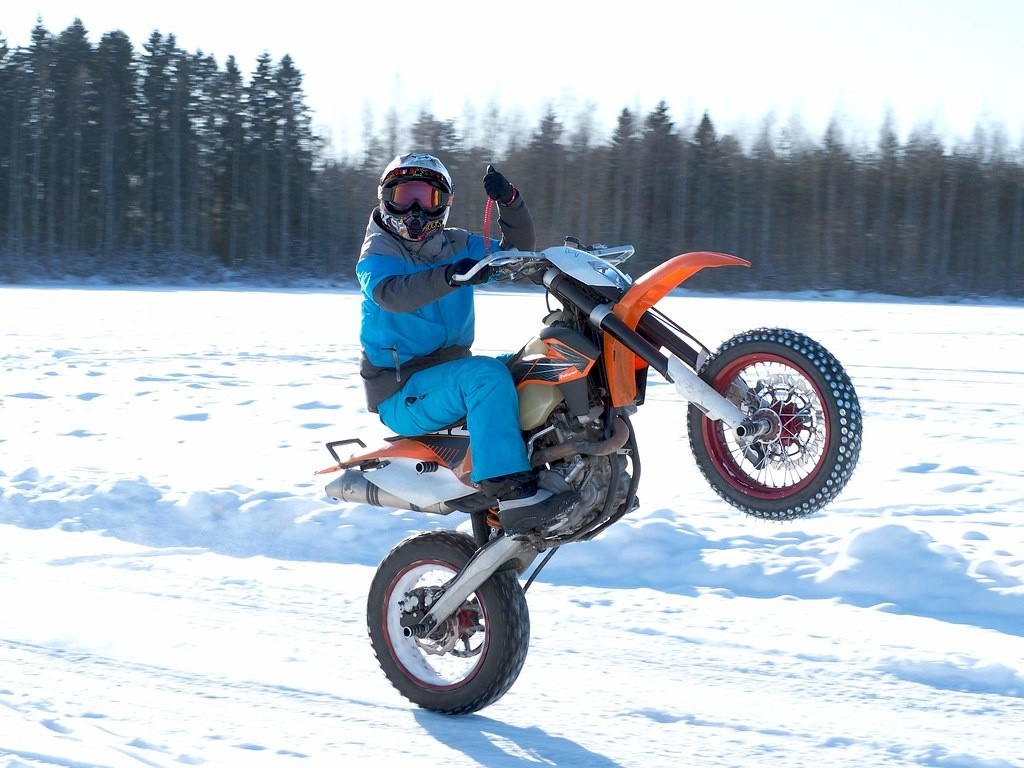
[502,183,515,207]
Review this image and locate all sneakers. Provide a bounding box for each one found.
[496,487,582,540]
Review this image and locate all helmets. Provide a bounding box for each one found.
[378,152,454,242]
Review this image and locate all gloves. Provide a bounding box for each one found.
[444,257,492,288]
[483,164,515,204]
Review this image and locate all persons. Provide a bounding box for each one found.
[355,153,578,537]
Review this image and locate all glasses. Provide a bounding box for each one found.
[385,180,449,217]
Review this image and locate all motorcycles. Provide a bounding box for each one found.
[312,238,865,716]
